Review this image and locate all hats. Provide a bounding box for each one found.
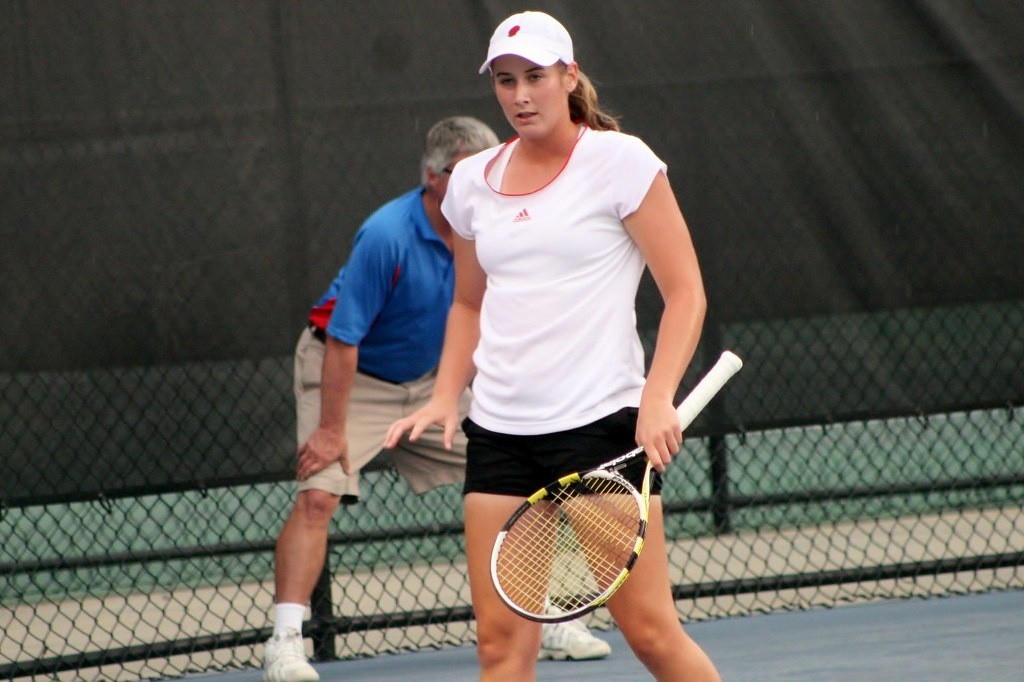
[479,11,574,74]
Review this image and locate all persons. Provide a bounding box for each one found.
[381,8,727,682]
[261,116,614,682]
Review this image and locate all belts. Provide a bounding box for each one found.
[308,320,326,345]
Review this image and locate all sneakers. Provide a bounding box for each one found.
[537,606,611,661]
[262,626,320,682]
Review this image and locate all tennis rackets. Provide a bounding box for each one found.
[488,349,743,623]
